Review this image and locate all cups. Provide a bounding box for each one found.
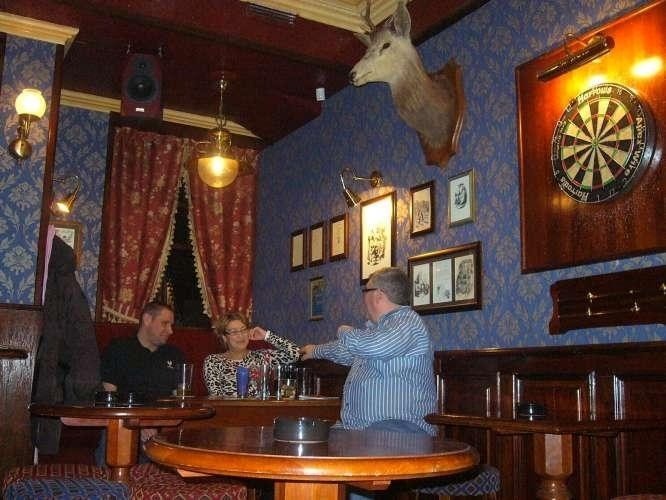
[234,360,322,403]
[175,361,194,401]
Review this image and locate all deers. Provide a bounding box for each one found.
[347,0,464,168]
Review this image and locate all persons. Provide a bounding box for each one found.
[78,300,188,467]
[200,310,302,397]
[297,266,442,440]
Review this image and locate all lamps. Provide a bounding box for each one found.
[5,84,49,163]
[532,28,618,87]
[191,65,240,194]
[336,162,385,209]
[45,173,85,219]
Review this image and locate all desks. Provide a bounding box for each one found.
[144,423,480,496]
[428,403,659,496]
[28,397,215,482]
[0,388,666,500]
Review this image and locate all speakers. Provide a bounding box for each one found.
[120,50,162,128]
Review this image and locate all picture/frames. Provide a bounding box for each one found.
[405,239,483,317]
[357,190,397,290]
[307,220,330,269]
[327,212,350,263]
[289,227,310,273]
[446,165,477,230]
[407,178,439,240]
[306,273,327,323]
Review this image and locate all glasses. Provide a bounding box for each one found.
[225,326,249,336]
[359,285,377,295]
